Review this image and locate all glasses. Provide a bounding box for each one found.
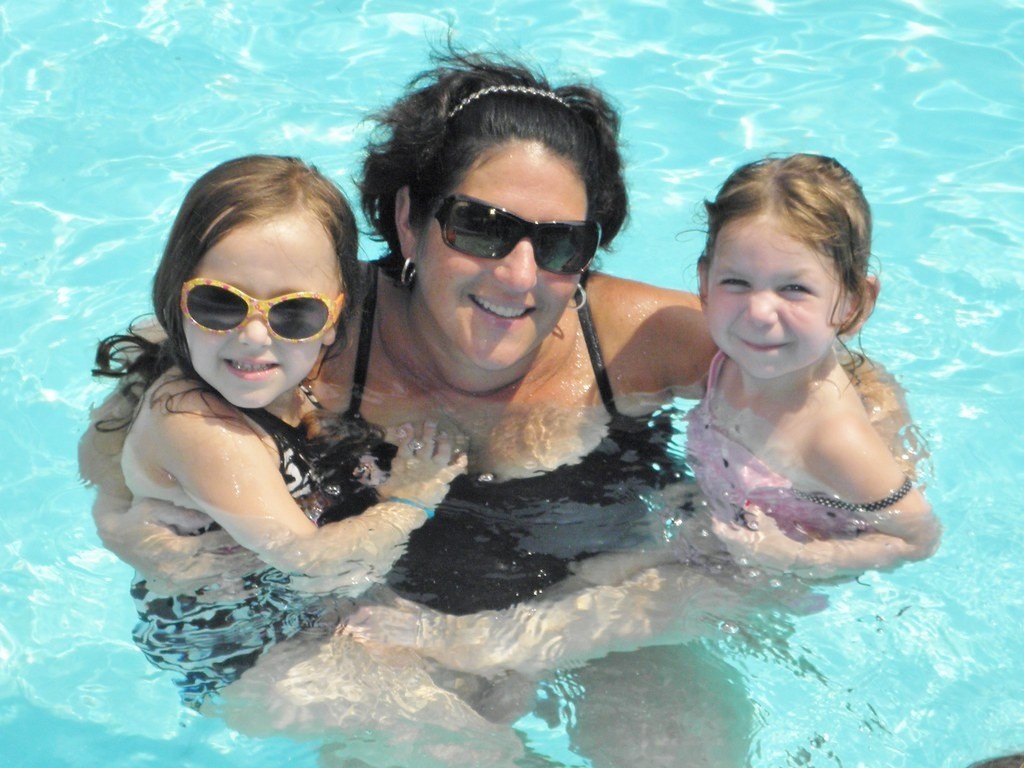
[180,278,345,344]
[423,192,601,275]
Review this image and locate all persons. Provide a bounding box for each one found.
[697,155,940,513]
[91,159,523,547]
[70,47,925,403]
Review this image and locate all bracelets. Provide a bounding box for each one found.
[383,497,439,520]
